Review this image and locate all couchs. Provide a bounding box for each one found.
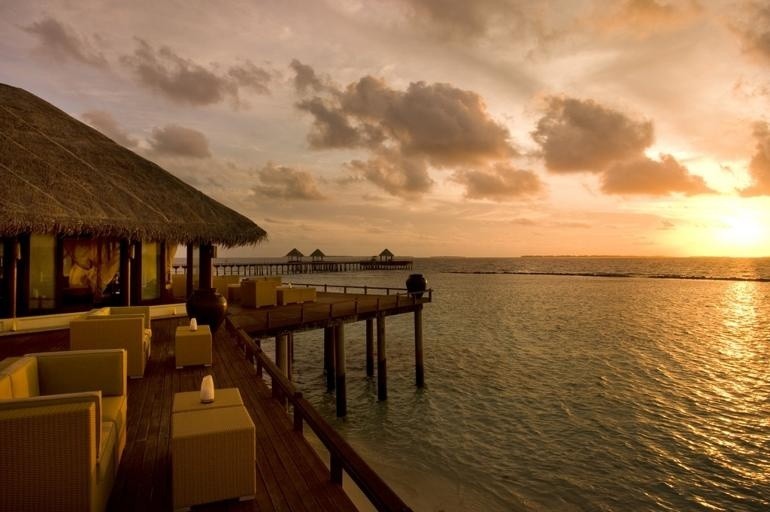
[69,306,152,380]
[1,349,131,512]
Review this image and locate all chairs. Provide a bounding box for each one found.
[240,280,278,308]
